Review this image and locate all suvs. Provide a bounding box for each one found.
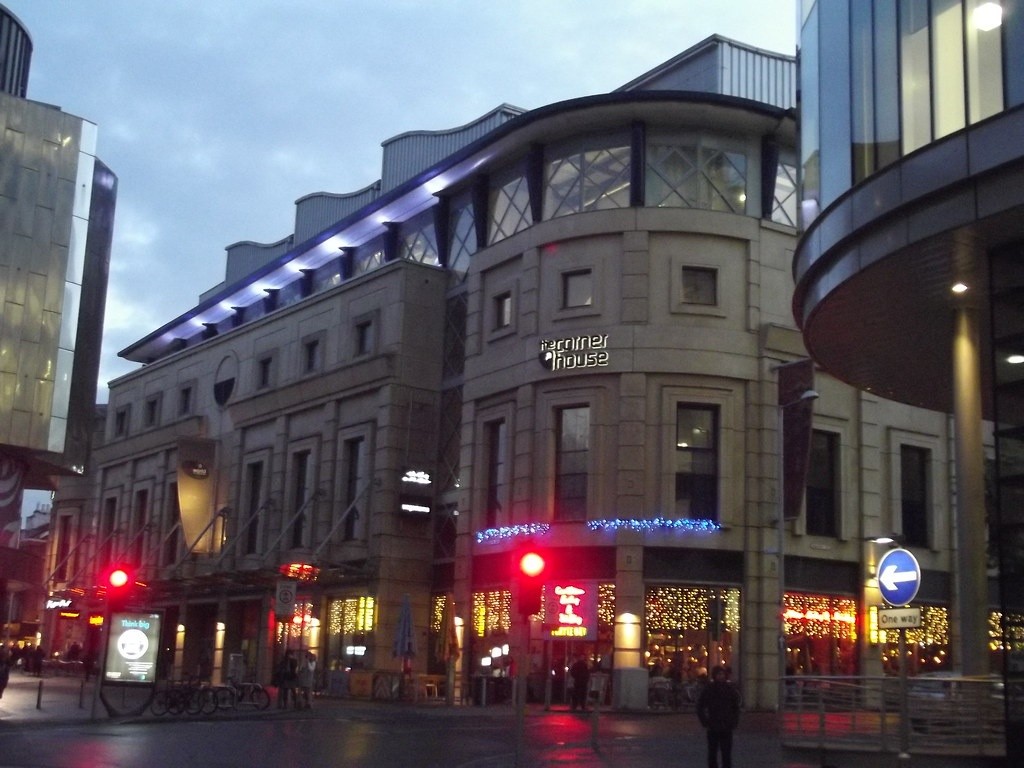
[910,672,1023,734]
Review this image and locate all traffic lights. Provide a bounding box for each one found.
[517,548,547,616]
[104,561,132,610]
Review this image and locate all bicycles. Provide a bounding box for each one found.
[149,678,311,717]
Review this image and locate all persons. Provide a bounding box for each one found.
[276,646,317,709]
[570,655,589,713]
[695,665,741,767]
[0,637,81,696]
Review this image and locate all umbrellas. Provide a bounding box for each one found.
[434,591,460,707]
[390,592,418,699]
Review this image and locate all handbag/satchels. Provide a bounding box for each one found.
[271,669,283,686]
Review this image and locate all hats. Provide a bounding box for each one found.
[712,665,725,679]
